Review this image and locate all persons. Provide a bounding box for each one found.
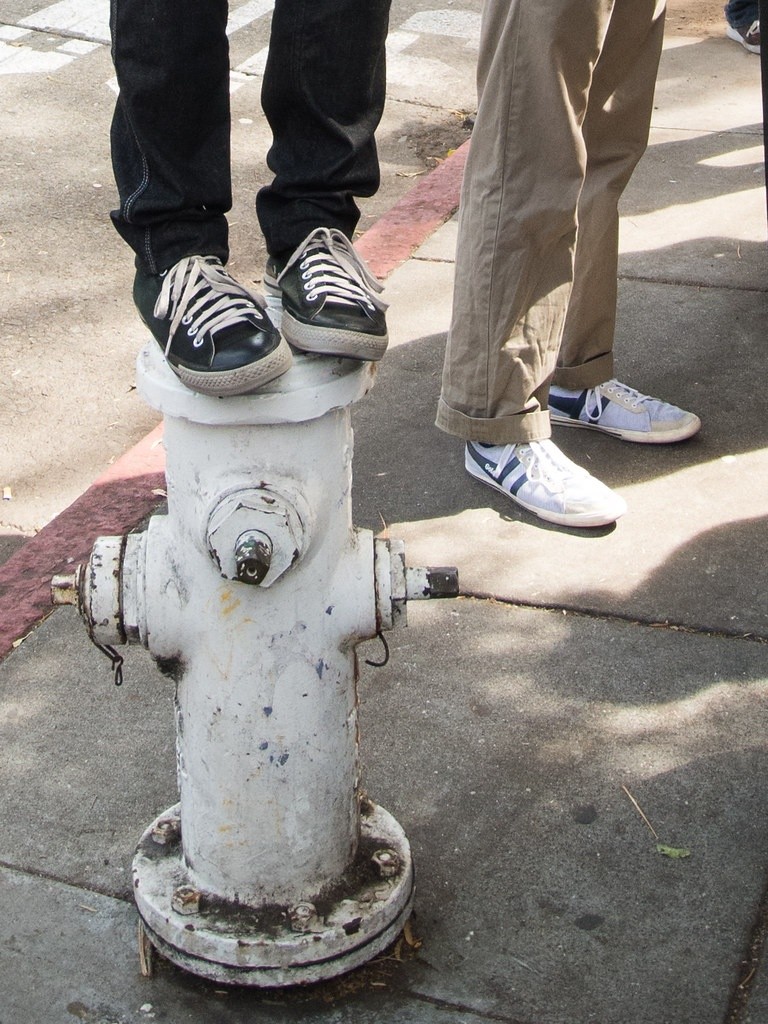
[433,0,703,532]
[723,0,761,56]
[106,1,388,397]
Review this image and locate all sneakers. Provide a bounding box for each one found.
[727,18,761,54]
[262,226,390,359]
[546,371,701,442]
[134,249,294,397]
[464,424,625,527]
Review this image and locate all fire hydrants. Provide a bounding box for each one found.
[52,289,460,990]
